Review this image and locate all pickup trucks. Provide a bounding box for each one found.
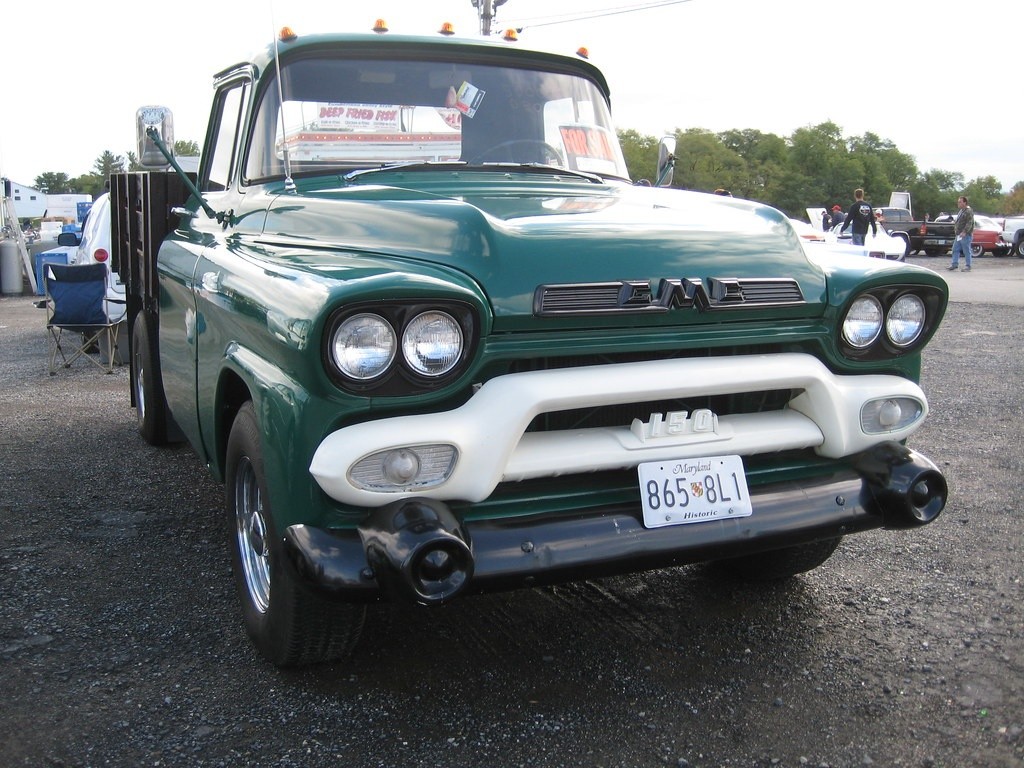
[1024,195,1024,258]
[104,25,958,640]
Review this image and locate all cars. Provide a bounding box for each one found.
[934,211,1006,256]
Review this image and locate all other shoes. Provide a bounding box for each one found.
[961,267,970,272]
[946,266,958,271]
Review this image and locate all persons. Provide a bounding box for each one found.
[820,212,833,233]
[947,196,975,273]
[831,204,844,232]
[840,188,876,246]
[912,214,932,253]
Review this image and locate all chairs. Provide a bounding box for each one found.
[42,261,127,379]
